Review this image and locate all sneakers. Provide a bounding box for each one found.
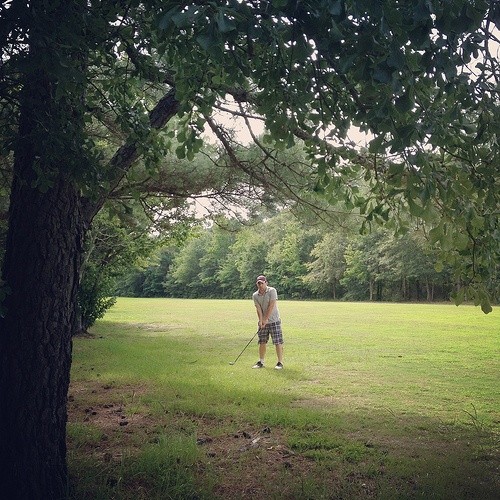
[251,361,263,368]
[274,362,283,369]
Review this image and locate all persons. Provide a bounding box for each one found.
[252,275,285,370]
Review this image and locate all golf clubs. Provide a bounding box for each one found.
[229,329,261,365]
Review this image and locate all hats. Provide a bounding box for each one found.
[256,276,266,283]
